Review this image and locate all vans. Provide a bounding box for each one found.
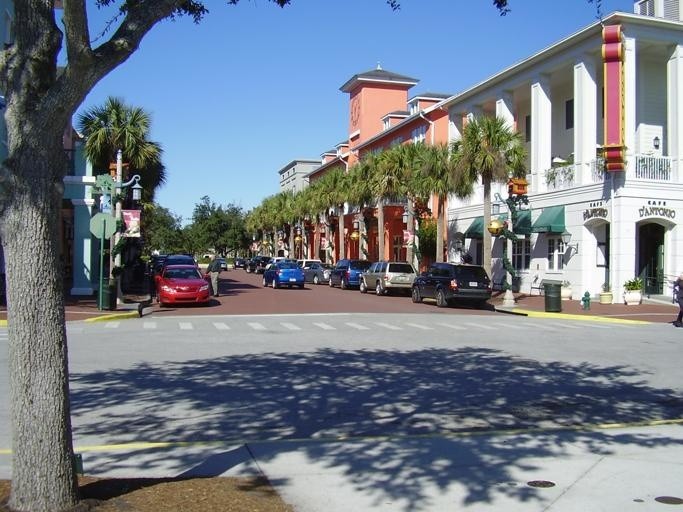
[233,256,494,307]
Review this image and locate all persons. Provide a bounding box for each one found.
[672,274,682,327]
[204,254,221,297]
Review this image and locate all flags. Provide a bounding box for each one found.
[401,228,414,249]
[118,208,141,238]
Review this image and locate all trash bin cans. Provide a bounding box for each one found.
[543,282,564,312]
[102,277,117,310]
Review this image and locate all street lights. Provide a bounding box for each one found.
[489,192,515,307]
[114,149,143,305]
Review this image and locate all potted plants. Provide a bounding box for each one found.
[652,135,661,151]
[622,276,645,308]
[598,285,614,306]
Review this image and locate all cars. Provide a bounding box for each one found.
[150,255,210,307]
[216,258,228,271]
